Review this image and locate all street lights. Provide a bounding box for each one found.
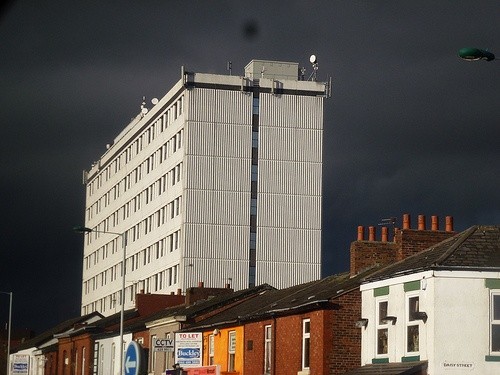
[0,291,14,375]
[71,225,128,375]
